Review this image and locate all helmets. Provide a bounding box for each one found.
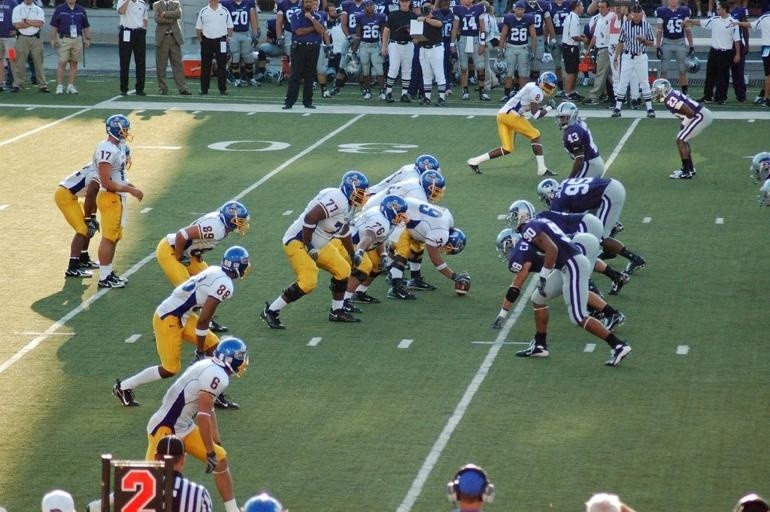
[492,61,508,75]
[380,194,408,225]
[509,199,535,232]
[340,170,370,207]
[344,62,361,79]
[105,114,134,144]
[651,79,672,103]
[555,101,578,131]
[419,170,445,201]
[219,200,250,235]
[124,142,132,170]
[415,155,440,174]
[685,57,701,74]
[439,227,466,256]
[242,494,288,512]
[212,338,249,378]
[537,178,559,208]
[495,228,522,264]
[537,71,558,96]
[757,179,770,207]
[222,246,251,280]
[750,151,770,185]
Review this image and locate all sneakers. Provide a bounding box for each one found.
[111,378,140,407]
[611,108,622,117]
[603,310,625,331]
[136,92,146,96]
[500,95,509,102]
[760,99,770,106]
[214,393,240,410]
[647,109,655,117]
[328,308,362,323]
[259,301,287,329]
[467,158,483,175]
[419,97,431,105]
[610,221,624,237]
[669,169,692,179]
[80,256,99,268]
[65,264,92,277]
[304,104,316,110]
[180,89,192,95]
[582,99,599,106]
[717,99,726,105]
[463,93,470,100]
[55,85,64,95]
[608,272,631,296]
[620,256,646,275]
[10,86,19,92]
[209,320,228,333]
[479,92,491,101]
[248,79,260,87]
[515,340,549,357]
[111,271,128,283]
[563,92,585,102]
[673,167,696,175]
[40,86,50,92]
[351,291,379,304]
[753,95,763,104]
[120,92,128,96]
[234,79,242,87]
[385,92,394,102]
[343,298,362,314]
[97,275,125,288]
[385,273,407,285]
[378,92,385,101]
[363,93,372,100]
[604,342,632,366]
[66,83,79,94]
[407,277,436,291]
[400,94,411,102]
[435,98,446,106]
[282,104,293,109]
[537,167,558,177]
[387,286,416,300]
[696,96,713,104]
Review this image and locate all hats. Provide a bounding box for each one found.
[157,435,184,455]
[632,4,642,11]
[41,489,75,512]
[512,1,526,9]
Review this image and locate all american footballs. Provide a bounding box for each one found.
[454,271,471,296]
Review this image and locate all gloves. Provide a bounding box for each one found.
[205,452,217,473]
[656,47,663,60]
[251,36,259,48]
[276,39,283,49]
[688,47,696,58]
[304,242,319,262]
[191,349,206,364]
[84,218,95,237]
[544,99,556,112]
[529,48,536,60]
[537,267,552,297]
[379,253,388,271]
[91,213,100,233]
[492,308,508,328]
[451,272,471,284]
[351,249,364,267]
[550,39,557,51]
[680,118,687,129]
[178,255,191,267]
[496,48,505,60]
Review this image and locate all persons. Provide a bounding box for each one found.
[326,3,339,29]
[362,170,445,213]
[493,228,626,333]
[364,154,440,195]
[276,0,304,56]
[282,0,325,110]
[450,0,492,101]
[0,0,50,94]
[686,2,741,107]
[498,0,536,103]
[448,463,496,512]
[561,1,588,102]
[611,5,655,118]
[112,246,251,408]
[544,0,571,90]
[468,72,558,176]
[156,201,250,288]
[255,3,287,85]
[222,0,261,87]
[534,210,631,296]
[729,13,770,107]
[750,152,770,208]
[387,197,471,300]
[117,0,148,95]
[85,337,249,512]
[342,194,408,313]
[352,0,385,101]
[55,143,130,277]
[650,78,713,179]
[432,1,453,97]
[480,1,498,91]
[95,114,143,288]
[555,102,604,179]
[712,3,749,104]
[50,0,91,95]
[195,0,234,96]
[654,1,695,96]
[520,0,556,75]
[606,6,641,110]
[259,171,369,330]
[382,0,414,102]
[509,199,632,367]
[589,12,600,34]
[411,1,446,106]
[152,0,192,95]
[312,0,330,98]
[536,177,647,276]
[154,434,213,512]
[585,492,636,512]
[329,0,367,96]
[584,0,613,105]
[587,0,599,15]
